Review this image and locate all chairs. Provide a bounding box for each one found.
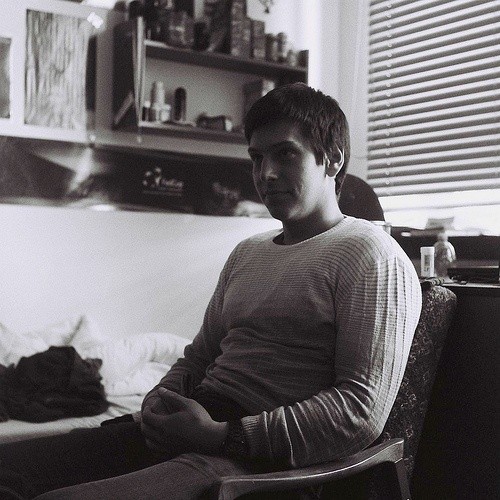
[208,280,458,500]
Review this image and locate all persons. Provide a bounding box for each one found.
[0,83,422,500]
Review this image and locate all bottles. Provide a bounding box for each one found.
[151,80,166,106]
[434,233,456,277]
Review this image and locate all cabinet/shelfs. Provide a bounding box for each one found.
[109,16,310,143]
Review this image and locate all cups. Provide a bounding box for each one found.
[174,85,187,122]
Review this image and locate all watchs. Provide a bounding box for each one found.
[227,413,250,467]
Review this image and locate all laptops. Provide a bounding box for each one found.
[447,265,499,282]
